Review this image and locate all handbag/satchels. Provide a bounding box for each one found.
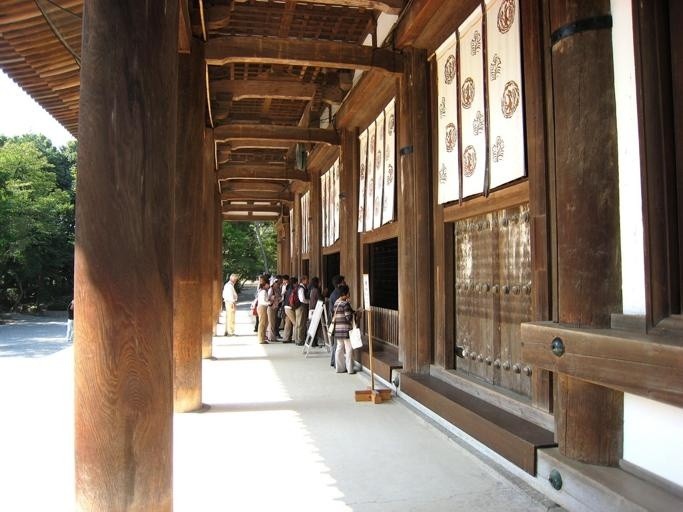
[347,313,364,350]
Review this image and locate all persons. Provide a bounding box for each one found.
[222,274,238,336]
[67,300,75,341]
[252,275,358,373]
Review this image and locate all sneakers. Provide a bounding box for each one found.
[252,323,319,348]
[223,331,236,337]
[330,362,356,375]
